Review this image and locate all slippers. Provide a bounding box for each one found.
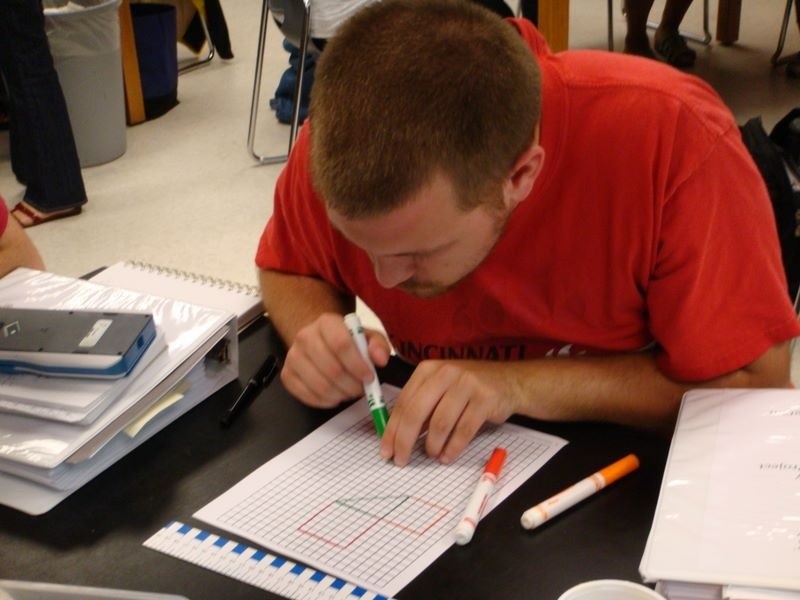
[11,200,85,226]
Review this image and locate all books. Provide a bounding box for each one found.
[88,258,266,333]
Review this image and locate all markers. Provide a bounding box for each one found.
[345,312,389,438]
[519,455,640,530]
[454,447,508,545]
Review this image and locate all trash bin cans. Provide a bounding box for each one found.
[43,0,127,167]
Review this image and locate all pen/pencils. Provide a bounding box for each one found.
[228,353,283,415]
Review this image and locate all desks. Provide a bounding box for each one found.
[0,266,679,600]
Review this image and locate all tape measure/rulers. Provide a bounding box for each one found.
[141,520,395,600]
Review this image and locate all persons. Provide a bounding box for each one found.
[625,0,695,71]
[0,0,88,227]
[256,0,800,466]
[276,0,378,126]
[0,200,44,278]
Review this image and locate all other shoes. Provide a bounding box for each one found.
[622,42,661,61]
[655,29,699,69]
